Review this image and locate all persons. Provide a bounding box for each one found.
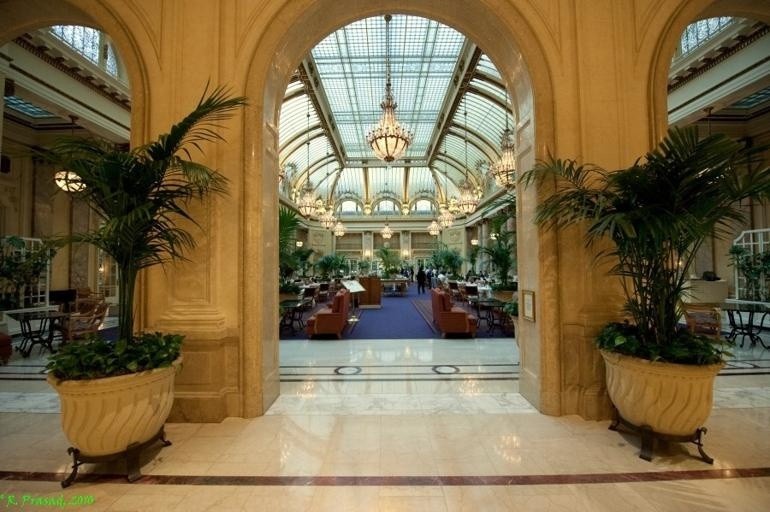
[401,266,496,295]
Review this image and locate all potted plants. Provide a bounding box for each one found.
[522,121,767,471]
[47,77,244,488]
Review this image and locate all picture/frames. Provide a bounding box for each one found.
[521,289,535,322]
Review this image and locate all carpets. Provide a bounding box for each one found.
[412,299,499,337]
[283,301,362,335]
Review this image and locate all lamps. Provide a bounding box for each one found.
[366,14,413,164]
[297,95,318,220]
[458,89,513,219]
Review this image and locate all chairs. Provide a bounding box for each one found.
[677,297,720,341]
[55,288,108,345]
[303,283,329,303]
[448,281,478,304]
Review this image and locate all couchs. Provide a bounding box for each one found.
[306,288,351,339]
[431,288,475,339]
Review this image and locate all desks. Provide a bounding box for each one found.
[279,299,312,334]
[720,298,769,349]
[6,310,67,357]
[467,294,506,335]
